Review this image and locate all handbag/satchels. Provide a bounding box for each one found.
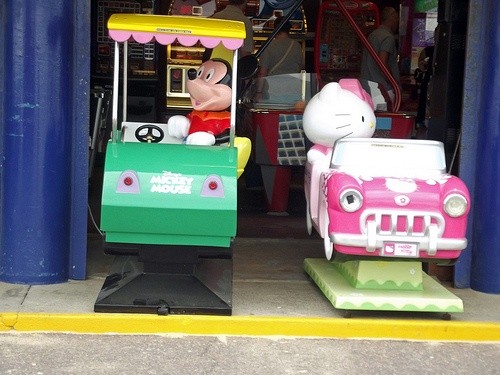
[414,68,431,87]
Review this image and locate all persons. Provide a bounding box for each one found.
[414,27,438,140]
[210,0,254,63]
[360,7,403,113]
[253,15,305,103]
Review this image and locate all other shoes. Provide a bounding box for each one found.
[415,123,420,130]
